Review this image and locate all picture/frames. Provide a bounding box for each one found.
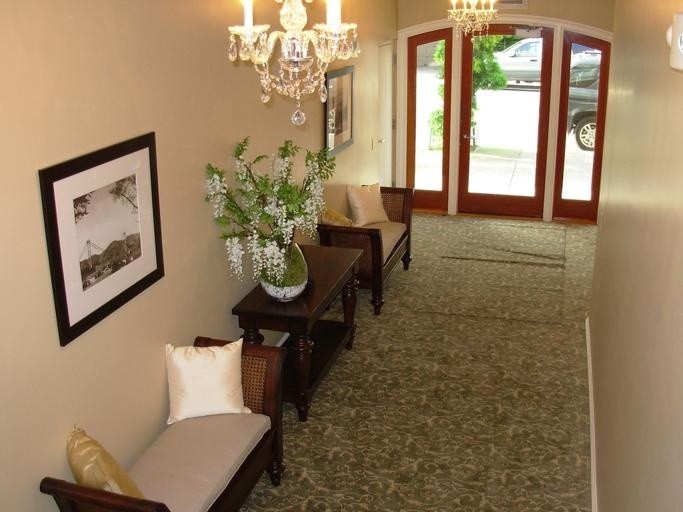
[38,130,165,348]
[322,65,355,160]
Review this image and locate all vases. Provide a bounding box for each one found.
[260,242,310,302]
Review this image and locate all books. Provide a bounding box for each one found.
[258,329,290,348]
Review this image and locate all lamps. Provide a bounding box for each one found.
[226,1,362,125]
[446,0,499,46]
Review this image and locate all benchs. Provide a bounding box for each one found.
[316,185,415,316]
[38,333,289,512]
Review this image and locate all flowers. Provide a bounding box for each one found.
[202,132,339,288]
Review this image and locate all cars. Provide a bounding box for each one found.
[484,37,542,81]
[568,51,602,150]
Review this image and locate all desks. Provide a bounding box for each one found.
[232,244,364,422]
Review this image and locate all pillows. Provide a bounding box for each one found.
[65,422,145,500]
[321,182,389,229]
[162,337,252,427]
